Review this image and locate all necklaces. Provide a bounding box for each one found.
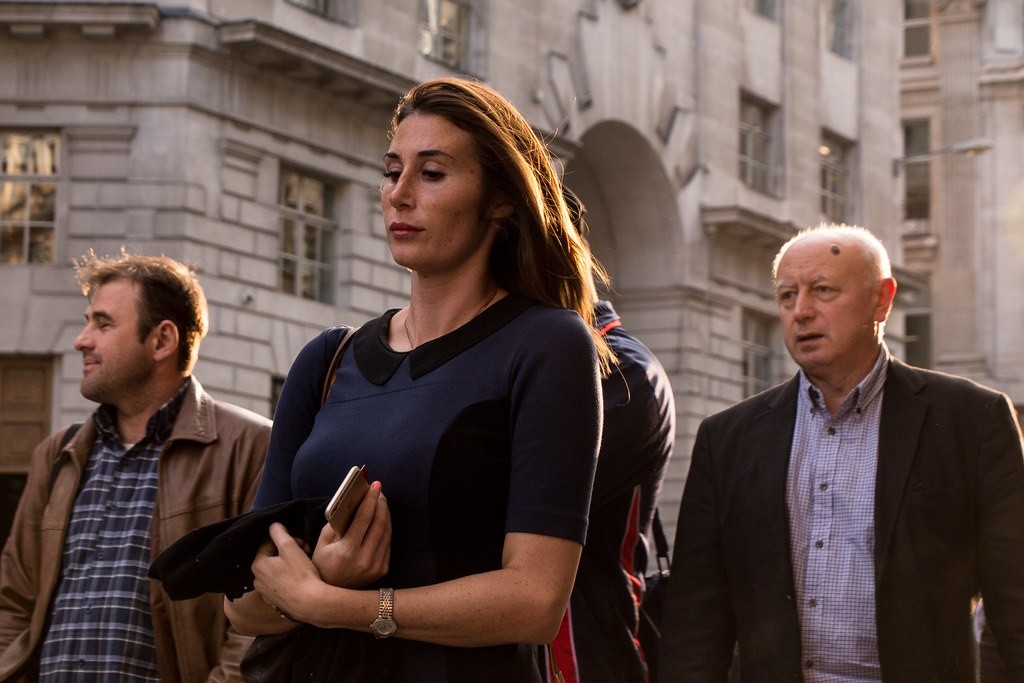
[402,289,497,349]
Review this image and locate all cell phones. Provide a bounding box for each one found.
[326,466,369,539]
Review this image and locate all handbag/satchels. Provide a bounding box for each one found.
[636,569,669,683]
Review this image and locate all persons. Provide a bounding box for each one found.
[1,247,273,682]
[223,76,605,683]
[656,223,1024,683]
[570,301,676,682]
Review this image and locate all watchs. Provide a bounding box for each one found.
[370,588,397,639]
